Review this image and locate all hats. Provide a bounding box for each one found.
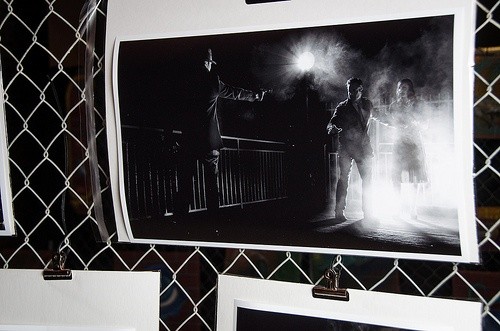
[197,48,216,65]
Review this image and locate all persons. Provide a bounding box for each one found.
[171,47,269,236]
[387,78,430,220]
[327,77,406,220]
